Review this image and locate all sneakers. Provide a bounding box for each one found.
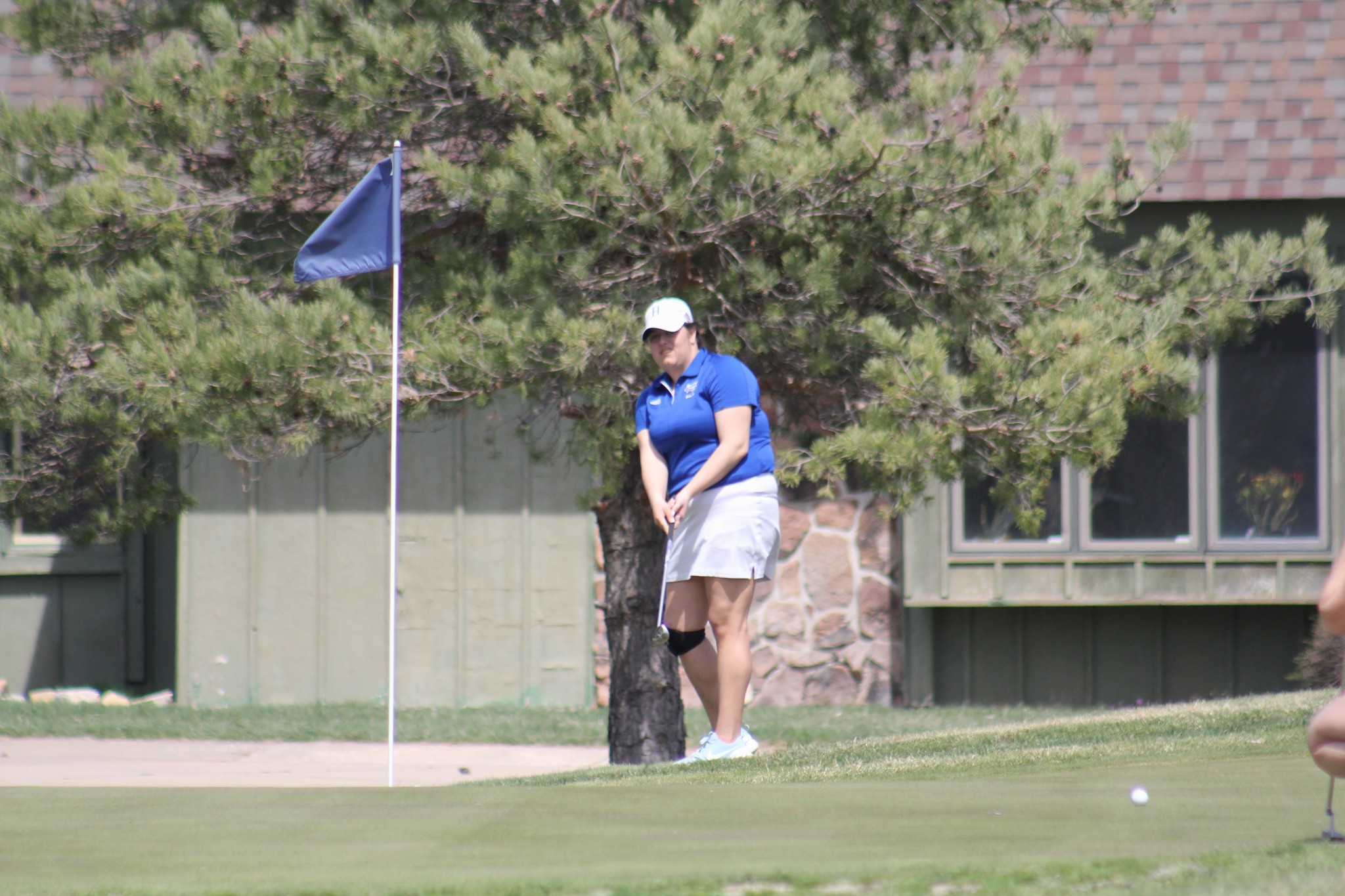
[740,725,758,754]
[672,730,753,766]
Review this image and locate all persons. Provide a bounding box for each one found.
[1304,545,1345,779]
[634,297,779,764]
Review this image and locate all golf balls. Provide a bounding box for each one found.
[1131,789,1149,807]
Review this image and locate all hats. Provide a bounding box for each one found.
[641,297,693,341]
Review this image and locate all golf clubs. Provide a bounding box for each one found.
[652,495,676,645]
[1322,649,1345,842]
